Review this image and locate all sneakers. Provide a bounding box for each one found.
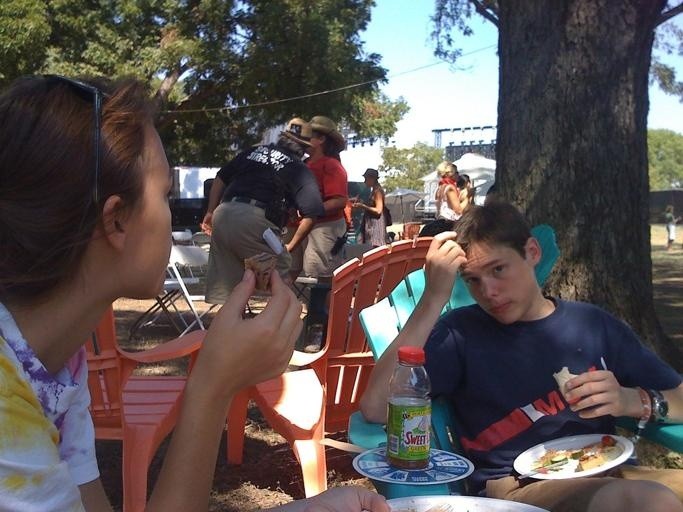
[303,324,325,352]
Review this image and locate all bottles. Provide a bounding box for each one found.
[386,345,432,467]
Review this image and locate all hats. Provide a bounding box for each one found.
[309,115,345,152]
[281,117,314,148]
[363,169,379,179]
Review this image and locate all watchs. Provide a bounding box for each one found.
[646,388,669,422]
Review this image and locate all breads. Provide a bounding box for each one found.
[553,367,580,404]
[577,452,605,472]
[245,253,277,290]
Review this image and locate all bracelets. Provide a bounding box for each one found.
[636,388,650,428]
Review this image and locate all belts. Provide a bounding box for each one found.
[220,196,267,210]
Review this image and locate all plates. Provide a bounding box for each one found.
[514,432,634,478]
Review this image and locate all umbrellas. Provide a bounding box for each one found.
[418,151,497,205]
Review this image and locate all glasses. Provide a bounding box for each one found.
[44,73,111,204]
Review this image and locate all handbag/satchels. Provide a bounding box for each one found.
[383,207,392,226]
[439,201,462,221]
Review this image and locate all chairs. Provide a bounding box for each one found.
[131,219,420,345]
[346,263,477,500]
[225,235,439,499]
[531,225,565,295]
[82,305,209,510]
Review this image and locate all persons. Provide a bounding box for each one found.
[0,71,388,512]
[201,115,347,350]
[348,169,387,247]
[360,201,683,511]
[664,205,681,251]
[436,162,472,222]
